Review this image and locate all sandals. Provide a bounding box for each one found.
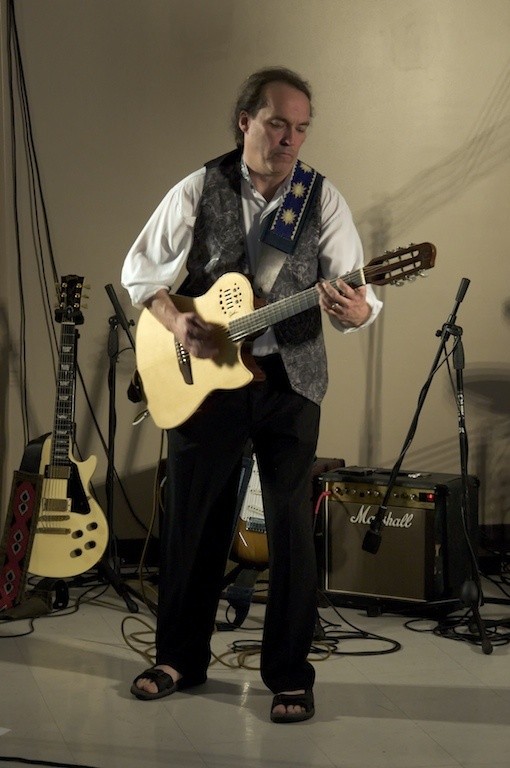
[270,685,316,723]
[131,661,209,701]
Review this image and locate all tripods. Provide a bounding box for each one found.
[43,283,142,614]
[366,278,510,654]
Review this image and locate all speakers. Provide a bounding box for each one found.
[321,464,480,615]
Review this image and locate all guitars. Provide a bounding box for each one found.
[26,270,114,580]
[134,241,437,433]
[228,456,269,570]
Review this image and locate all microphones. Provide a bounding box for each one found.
[362,508,385,553]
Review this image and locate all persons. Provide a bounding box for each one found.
[120,66,385,722]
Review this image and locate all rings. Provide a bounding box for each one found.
[330,302,340,309]
